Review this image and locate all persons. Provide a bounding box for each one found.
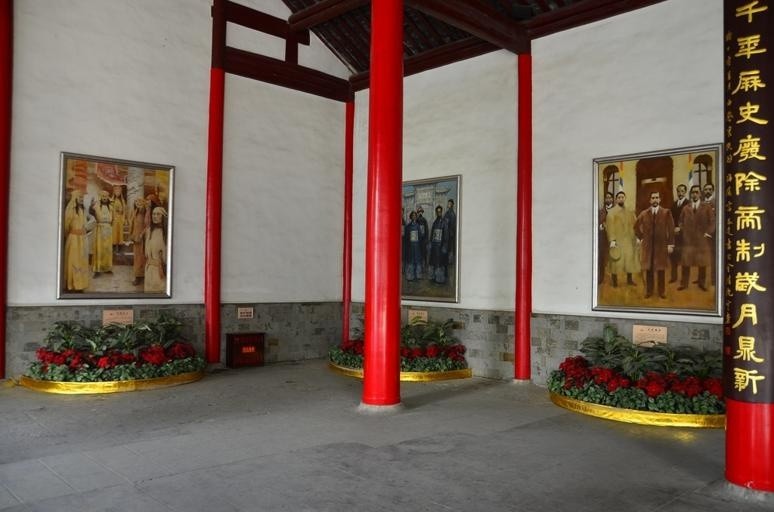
[64,185,167,293]
[598,184,715,298]
[402,199,456,284]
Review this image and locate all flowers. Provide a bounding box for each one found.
[323,303,473,376]
[17,307,207,387]
[539,323,728,419]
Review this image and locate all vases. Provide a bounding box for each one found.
[545,391,725,430]
[327,361,473,381]
[11,367,203,394]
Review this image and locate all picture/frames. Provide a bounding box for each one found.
[402,174,461,304]
[592,142,724,318]
[56,150,176,300]
